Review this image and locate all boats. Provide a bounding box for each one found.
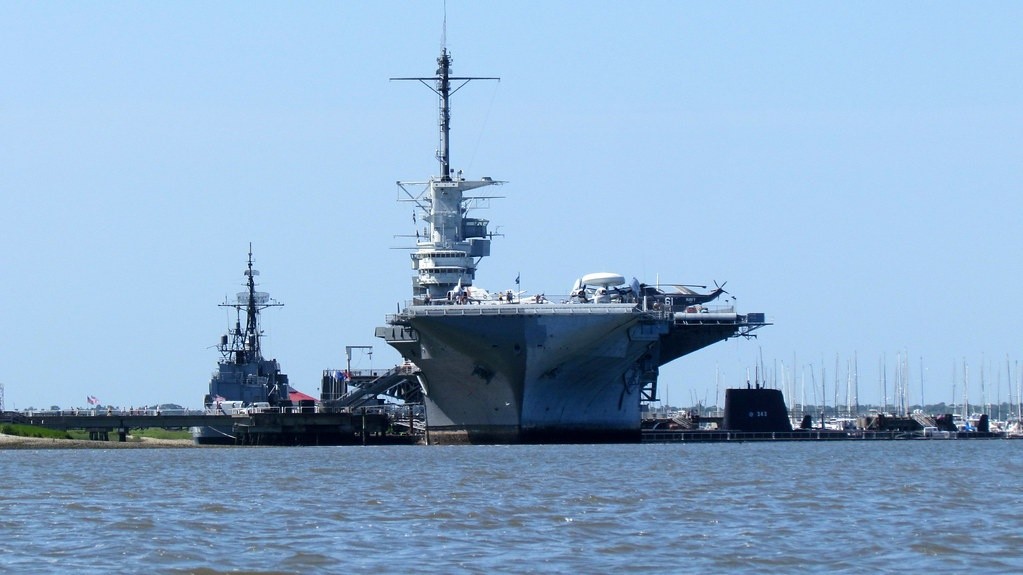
[191,238,299,459]
[644,345,1023,440]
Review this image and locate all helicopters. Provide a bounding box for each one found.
[635,278,729,312]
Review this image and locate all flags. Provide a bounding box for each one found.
[87,396,94,405]
[91,395,99,404]
[332,370,351,383]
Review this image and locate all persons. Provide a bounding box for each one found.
[55,404,162,416]
[452,289,473,305]
[695,301,702,313]
[217,401,254,416]
[535,293,569,305]
[423,294,430,305]
[325,367,329,376]
[394,364,398,373]
[498,290,513,304]
[386,369,390,378]
